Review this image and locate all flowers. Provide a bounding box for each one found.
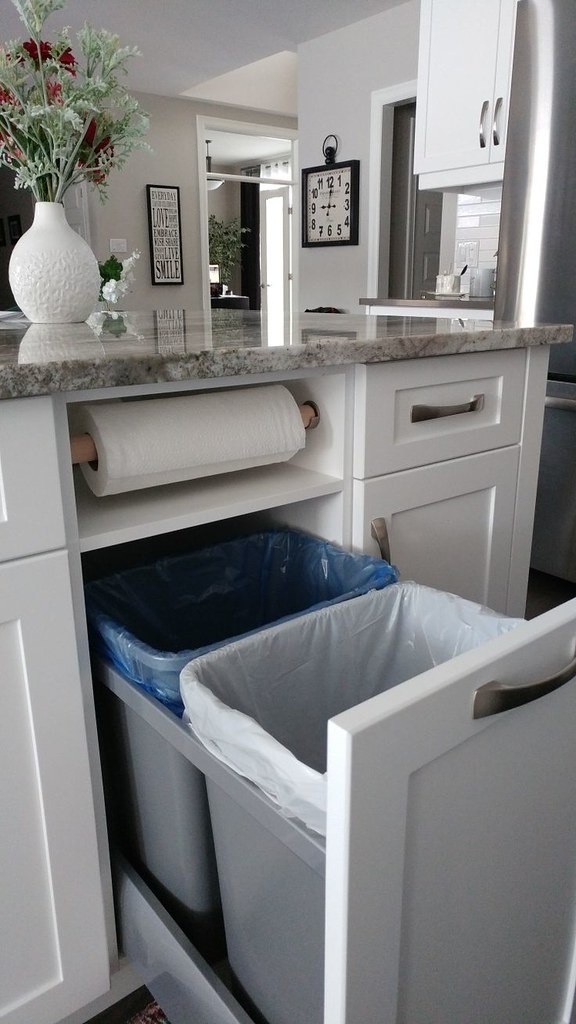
[98,248,144,311]
[0,0,158,205]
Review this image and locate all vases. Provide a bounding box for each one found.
[9,201,101,324]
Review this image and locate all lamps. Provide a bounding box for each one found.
[206,139,225,191]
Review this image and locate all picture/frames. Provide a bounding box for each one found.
[145,183,186,286]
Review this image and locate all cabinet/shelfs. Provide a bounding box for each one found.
[413,0,518,200]
[0,344,576,1024]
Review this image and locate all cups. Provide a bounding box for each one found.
[468,268,495,298]
[435,275,461,294]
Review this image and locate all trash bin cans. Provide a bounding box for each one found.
[87,518,531,1024]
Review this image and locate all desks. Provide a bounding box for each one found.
[211,295,249,309]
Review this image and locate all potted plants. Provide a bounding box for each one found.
[208,214,252,296]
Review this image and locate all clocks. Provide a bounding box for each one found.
[301,135,360,248]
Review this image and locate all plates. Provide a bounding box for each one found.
[427,291,464,300]
